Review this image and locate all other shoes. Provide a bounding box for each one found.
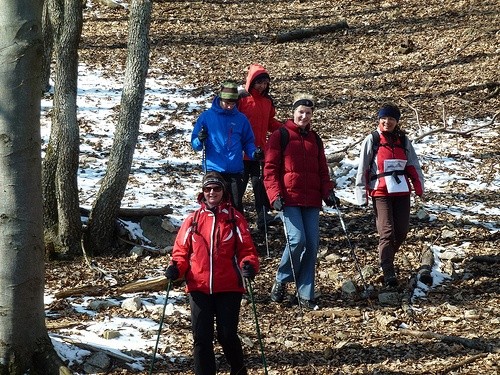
[385,276,399,286]
[270,279,286,303]
[257,214,275,231]
[294,291,320,310]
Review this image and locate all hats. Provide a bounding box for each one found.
[202,171,228,192]
[221,82,238,99]
[378,102,401,121]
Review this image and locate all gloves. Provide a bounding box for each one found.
[198,130,208,141]
[254,149,265,159]
[242,265,255,278]
[326,194,340,209]
[165,265,179,280]
[273,199,286,212]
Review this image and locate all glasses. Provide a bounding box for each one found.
[203,186,222,192]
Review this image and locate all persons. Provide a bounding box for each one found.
[355,102,424,290]
[240,62,285,216]
[191,80,265,217]
[263,92,340,310]
[165,170,259,375]
